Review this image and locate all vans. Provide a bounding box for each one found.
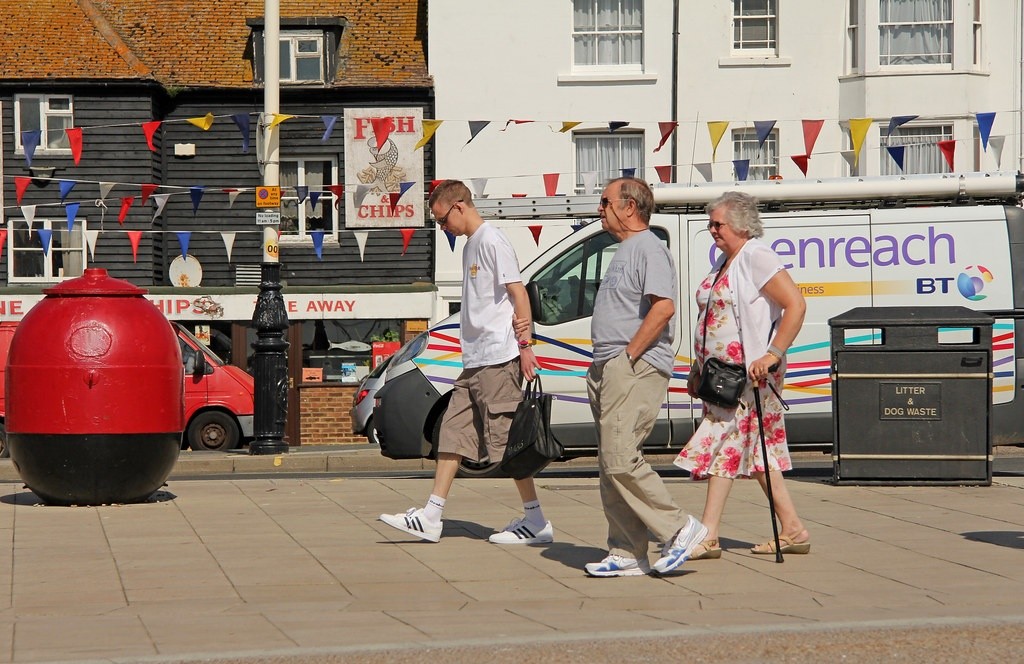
[372,199,1024,478]
[0,322,256,453]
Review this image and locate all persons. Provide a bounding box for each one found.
[380,179,555,545]
[585,177,708,577]
[672,191,811,561]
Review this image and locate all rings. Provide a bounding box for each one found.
[760,369,762,371]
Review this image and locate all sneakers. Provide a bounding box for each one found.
[584,553,652,577]
[488,517,554,545]
[653,516,707,573]
[379,509,444,542]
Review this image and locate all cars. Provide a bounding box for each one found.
[349,353,394,444]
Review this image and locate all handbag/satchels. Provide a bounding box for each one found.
[697,358,747,410]
[499,374,564,480]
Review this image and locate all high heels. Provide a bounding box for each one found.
[686,541,721,560]
[750,529,810,554]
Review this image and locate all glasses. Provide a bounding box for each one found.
[600,197,638,208]
[707,221,728,230]
[437,199,464,225]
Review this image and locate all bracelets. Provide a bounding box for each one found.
[518,339,532,348]
[767,345,784,360]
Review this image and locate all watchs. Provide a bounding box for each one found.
[626,353,635,364]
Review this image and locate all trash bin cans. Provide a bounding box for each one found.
[829,306,996,487]
